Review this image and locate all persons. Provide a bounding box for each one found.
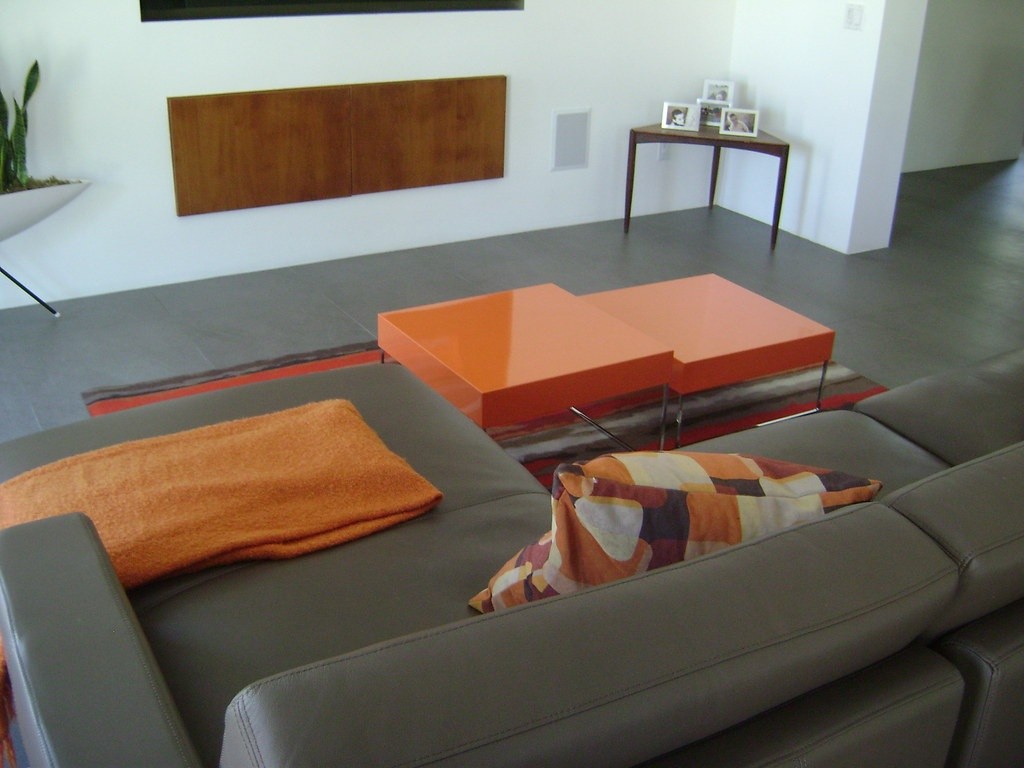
[729,114,749,134]
[700,90,727,124]
[670,110,684,127]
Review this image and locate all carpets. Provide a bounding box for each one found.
[82,342,889,498]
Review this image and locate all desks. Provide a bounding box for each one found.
[624,123,790,251]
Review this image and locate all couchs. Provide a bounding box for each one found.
[0,363,1024,768]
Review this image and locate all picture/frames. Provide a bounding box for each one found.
[661,102,701,131]
[704,79,735,107]
[697,98,730,126]
[718,108,760,138]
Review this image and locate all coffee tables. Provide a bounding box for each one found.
[377,275,835,452]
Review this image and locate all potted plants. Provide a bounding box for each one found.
[0,60,92,241]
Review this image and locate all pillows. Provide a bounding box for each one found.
[467,451,882,614]
